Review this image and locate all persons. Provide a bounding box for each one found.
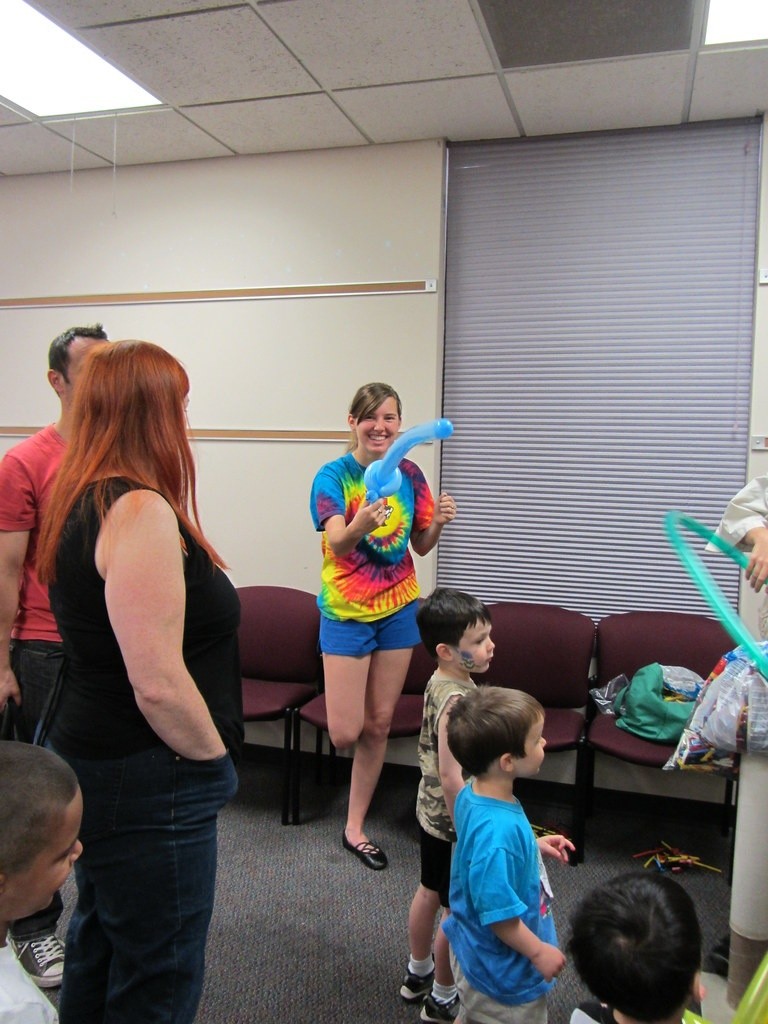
[0,740,83,1024]
[400,587,495,1023]
[704,475,768,642]
[0,323,111,988]
[310,382,456,871]
[568,871,706,1024]
[34,339,245,1024]
[440,685,575,1024]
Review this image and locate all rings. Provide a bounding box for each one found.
[378,509,382,513]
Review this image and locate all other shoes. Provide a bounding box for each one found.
[342,829,387,870]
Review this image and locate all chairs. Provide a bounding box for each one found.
[235,586,330,825]
[484,603,595,865]
[292,597,436,826]
[576,610,739,864]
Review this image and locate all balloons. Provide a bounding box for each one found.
[665,510,768,679]
[364,419,453,504]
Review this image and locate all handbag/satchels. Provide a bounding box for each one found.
[665,638,768,774]
[615,663,699,740]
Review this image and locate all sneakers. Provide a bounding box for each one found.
[399,951,436,999]
[5,928,68,987]
[421,987,460,1023]
